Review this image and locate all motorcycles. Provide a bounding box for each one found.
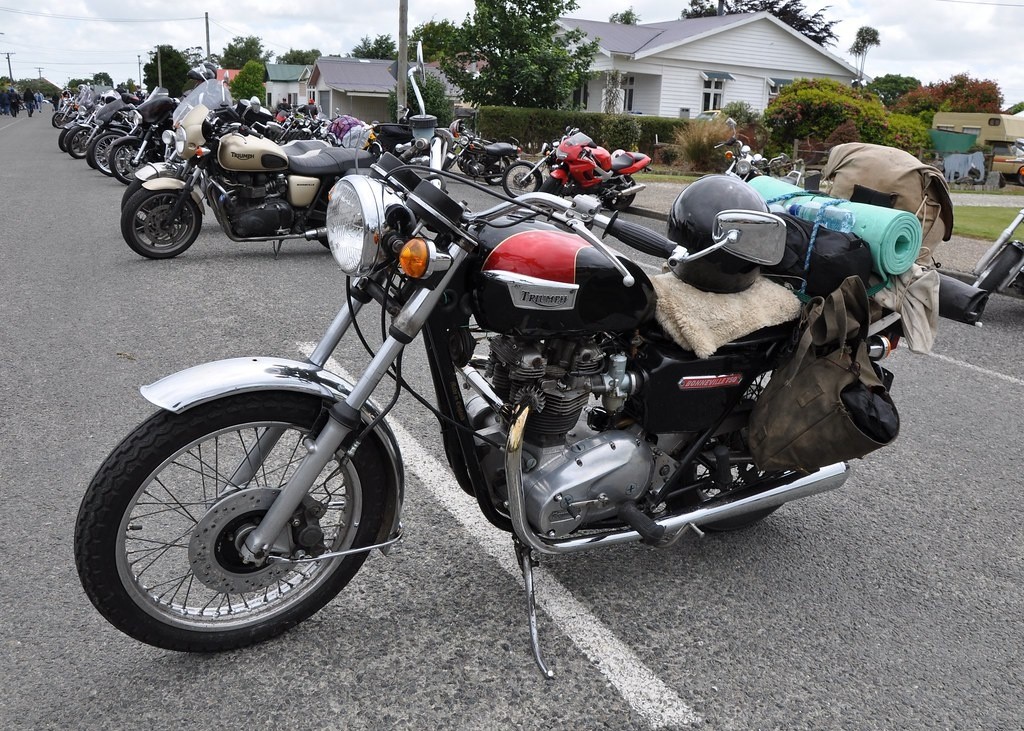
[275,106,332,143]
[714,117,802,186]
[120,63,374,260]
[51,82,272,185]
[442,124,522,185]
[971,206,1024,300]
[502,125,652,211]
[73,40,901,679]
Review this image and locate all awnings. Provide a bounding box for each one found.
[328,84,397,98]
[766,77,794,89]
[700,70,737,82]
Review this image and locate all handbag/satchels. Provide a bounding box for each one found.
[750,276,900,473]
[767,209,874,301]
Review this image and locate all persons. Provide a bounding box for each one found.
[276,97,319,126]
[0,87,64,118]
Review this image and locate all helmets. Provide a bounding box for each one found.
[666,174,771,294]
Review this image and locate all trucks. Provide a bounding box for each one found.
[932,112,1024,185]
